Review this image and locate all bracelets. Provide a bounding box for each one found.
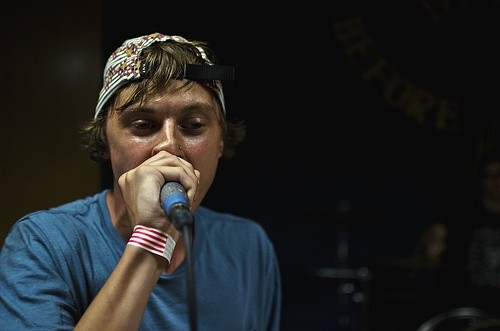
[128,224,175,268]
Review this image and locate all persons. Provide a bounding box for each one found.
[0,31,280,331]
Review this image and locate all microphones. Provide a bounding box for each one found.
[160,181,193,229]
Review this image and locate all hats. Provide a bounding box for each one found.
[93,31,227,124]
